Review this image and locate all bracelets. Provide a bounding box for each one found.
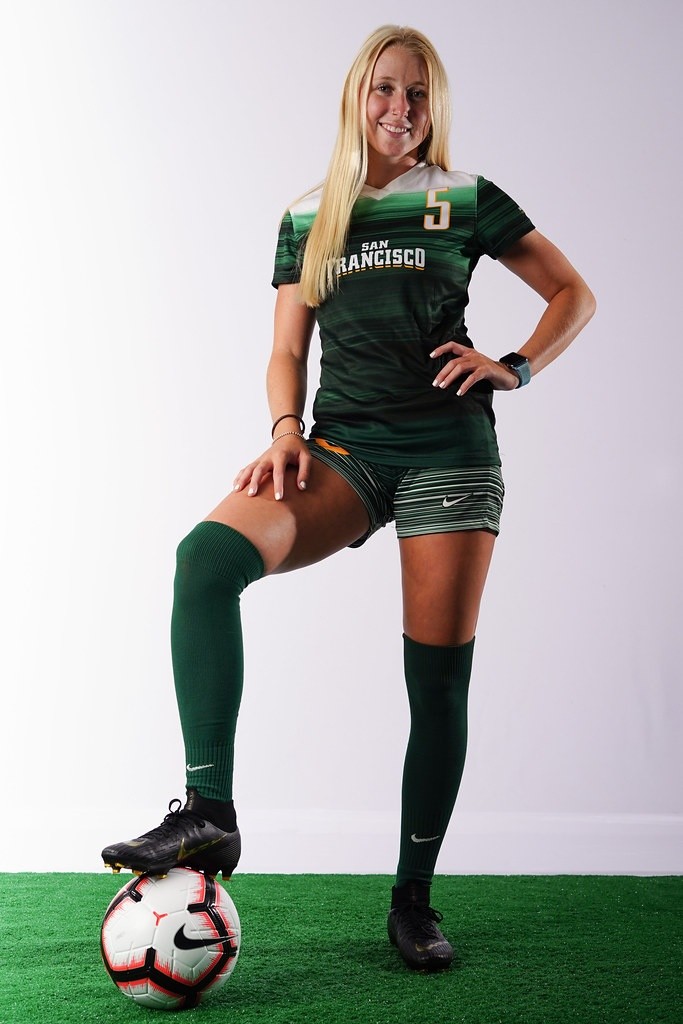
[271,414,304,437]
[272,431,304,443]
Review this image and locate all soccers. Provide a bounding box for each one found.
[100,867,241,1011]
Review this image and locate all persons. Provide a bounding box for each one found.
[98,27,598,973]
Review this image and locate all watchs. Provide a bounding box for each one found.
[497,352,531,390]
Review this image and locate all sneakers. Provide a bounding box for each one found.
[387,885,454,969]
[102,788,240,881]
